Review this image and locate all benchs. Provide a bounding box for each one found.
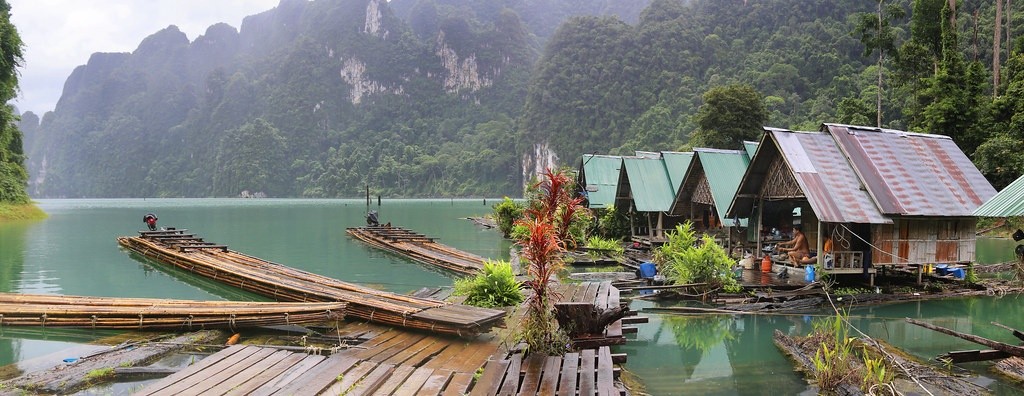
[147,234,193,242]
[167,241,215,249]
[359,226,406,231]
[366,229,412,236]
[388,235,440,243]
[159,238,202,246]
[380,233,425,240]
[139,229,187,238]
[373,231,417,239]
[177,245,228,254]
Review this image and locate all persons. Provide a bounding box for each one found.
[776,225,811,267]
[790,232,833,265]
